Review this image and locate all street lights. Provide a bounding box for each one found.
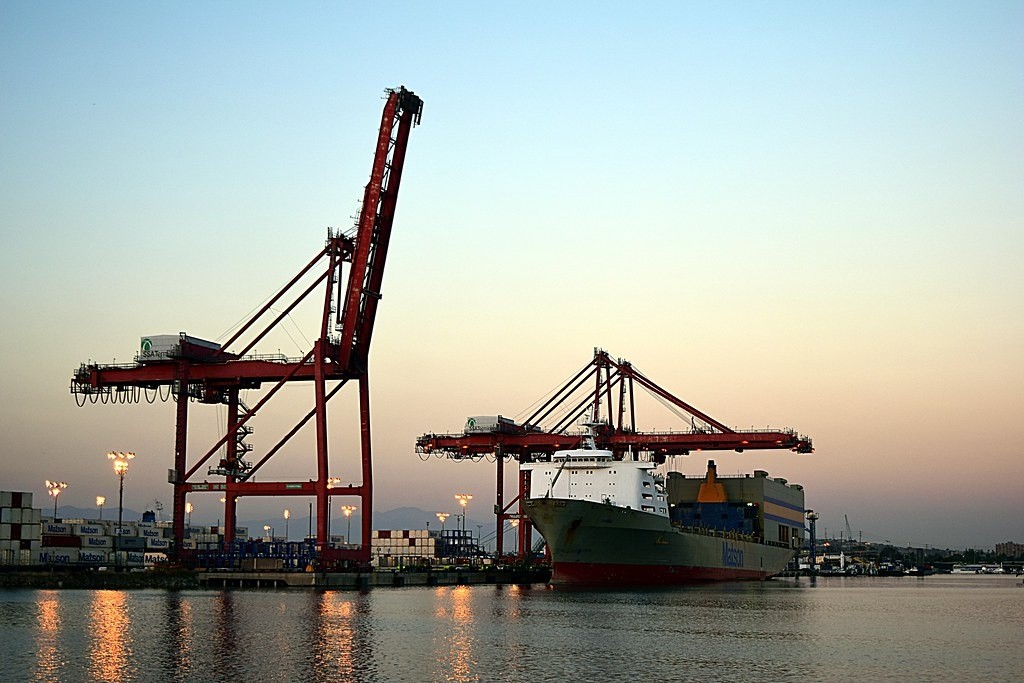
[436,512,450,529]
[108,449,135,539]
[95,496,106,520]
[455,494,472,531]
[342,504,358,546]
[45,479,68,521]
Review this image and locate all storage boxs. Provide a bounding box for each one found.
[466,415,515,433]
[0,492,484,575]
[140,335,219,364]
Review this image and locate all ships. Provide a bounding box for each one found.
[515,411,808,588]
